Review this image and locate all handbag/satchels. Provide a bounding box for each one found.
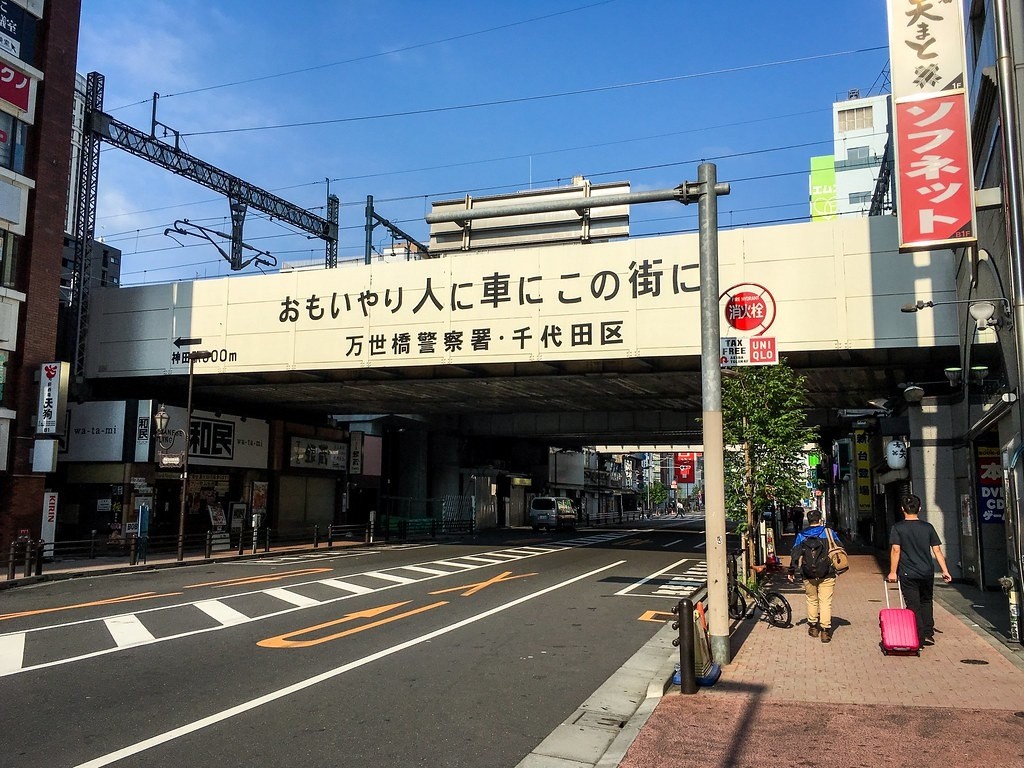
[825,528,849,575]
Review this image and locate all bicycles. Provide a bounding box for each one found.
[726,547,792,629]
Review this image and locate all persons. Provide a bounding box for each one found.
[787,510,843,643]
[791,501,805,540]
[637,500,643,511]
[674,500,684,519]
[888,494,952,650]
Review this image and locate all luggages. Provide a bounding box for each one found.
[879,577,920,657]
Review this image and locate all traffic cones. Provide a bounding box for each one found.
[764,536,779,570]
[695,600,710,638]
[672,609,721,687]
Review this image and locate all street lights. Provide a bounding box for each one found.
[153,350,214,561]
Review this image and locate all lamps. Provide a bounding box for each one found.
[945,367,965,387]
[882,397,899,410]
[970,366,990,386]
[904,386,925,402]
[900,297,1011,315]
[969,303,1000,330]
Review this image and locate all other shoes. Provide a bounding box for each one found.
[920,629,934,645]
[821,628,830,642]
[808,624,818,637]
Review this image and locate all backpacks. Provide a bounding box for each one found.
[801,536,836,580]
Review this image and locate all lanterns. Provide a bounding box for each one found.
[886,440,906,471]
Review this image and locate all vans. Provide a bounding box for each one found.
[530,496,576,533]
